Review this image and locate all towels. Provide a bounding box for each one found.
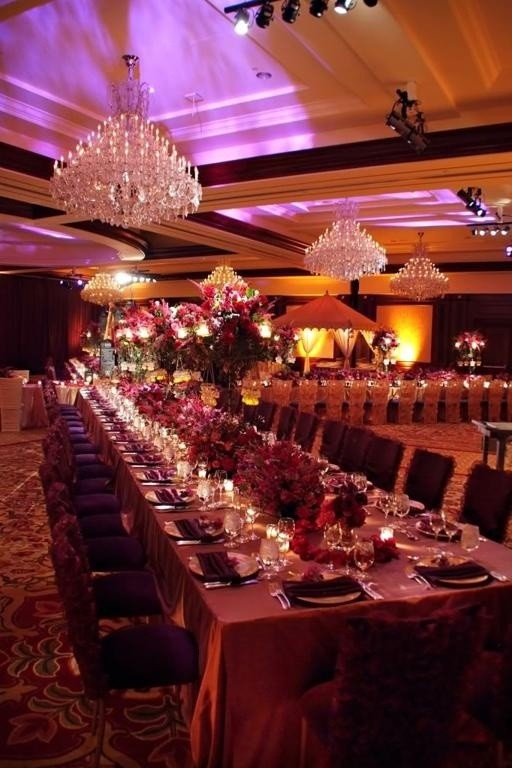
[142,469,171,482]
[100,404,111,410]
[133,454,155,464]
[174,518,213,541]
[102,410,115,415]
[343,473,375,491]
[98,399,107,404]
[107,416,120,423]
[154,488,186,505]
[112,423,123,431]
[383,494,424,516]
[283,575,364,599]
[116,433,133,441]
[90,385,101,400]
[410,505,461,538]
[125,441,144,452]
[415,560,487,582]
[198,552,241,580]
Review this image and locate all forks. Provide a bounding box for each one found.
[275,583,291,608]
[409,568,431,589]
[406,569,423,586]
[268,584,287,609]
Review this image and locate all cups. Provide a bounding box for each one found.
[430,510,445,547]
[324,521,341,570]
[260,538,280,581]
[278,518,297,567]
[395,495,409,525]
[353,471,367,493]
[444,517,457,548]
[223,512,240,548]
[265,523,279,542]
[198,484,210,512]
[207,473,220,509]
[340,528,357,576]
[216,470,228,507]
[197,452,209,484]
[380,492,393,527]
[354,540,374,581]
[461,525,480,558]
[245,496,261,541]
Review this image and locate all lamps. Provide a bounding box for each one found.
[491,227,500,237]
[505,245,512,258]
[255,6,275,28]
[479,228,490,237]
[51,55,202,225]
[471,226,476,235]
[334,0,354,14]
[305,195,388,281]
[309,1,332,16]
[362,0,378,8]
[390,234,449,301]
[457,188,476,208]
[235,10,250,35]
[466,196,487,218]
[199,258,246,299]
[81,266,124,305]
[500,225,510,237]
[282,1,300,24]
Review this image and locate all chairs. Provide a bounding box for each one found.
[468,380,484,421]
[295,412,316,453]
[49,502,130,537]
[46,482,119,515]
[507,382,512,423]
[49,428,97,452]
[56,418,86,433]
[299,381,319,412]
[326,381,343,420]
[39,465,115,492]
[370,378,388,424]
[256,400,277,432]
[487,379,502,422]
[215,384,255,424]
[277,406,297,440]
[49,441,101,464]
[397,379,416,424]
[65,594,194,766]
[320,421,341,461]
[404,449,453,512]
[50,539,164,626]
[48,453,113,478]
[336,426,374,471]
[445,379,462,422]
[361,435,400,492]
[270,378,293,406]
[56,401,82,421]
[53,425,91,442]
[459,459,512,543]
[50,511,143,578]
[422,380,441,424]
[346,379,366,423]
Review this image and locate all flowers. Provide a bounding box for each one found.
[176,405,225,442]
[371,327,399,373]
[173,286,299,398]
[271,322,300,373]
[132,383,174,418]
[117,380,132,396]
[187,424,270,480]
[157,397,211,427]
[451,331,486,374]
[277,464,369,531]
[234,444,312,513]
[122,384,144,400]
[98,298,206,371]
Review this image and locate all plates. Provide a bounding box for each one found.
[145,489,195,504]
[288,572,362,605]
[136,471,173,482]
[110,435,143,441]
[189,552,259,580]
[417,558,489,585]
[123,454,163,464]
[415,520,466,538]
[119,445,153,452]
[104,426,130,431]
[164,519,224,539]
[376,500,425,512]
